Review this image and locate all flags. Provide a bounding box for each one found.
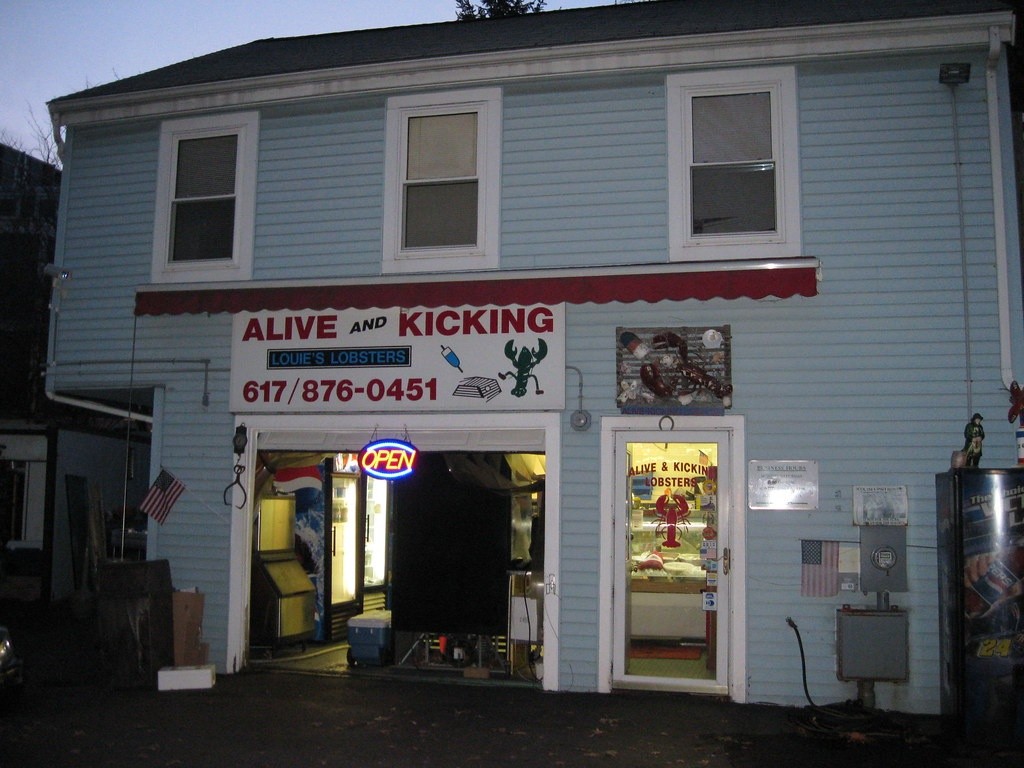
[139,469,185,525]
[801,538,840,597]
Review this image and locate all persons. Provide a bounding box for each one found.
[964,413,986,468]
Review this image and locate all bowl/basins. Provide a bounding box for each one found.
[663,562,690,575]
